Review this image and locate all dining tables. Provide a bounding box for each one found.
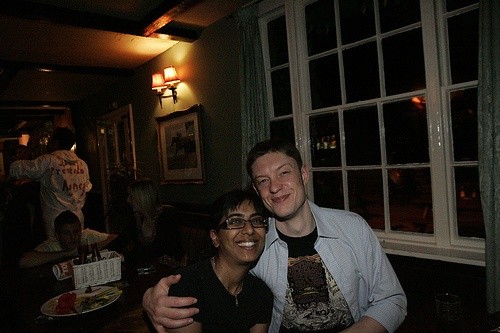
[0,257,176,333]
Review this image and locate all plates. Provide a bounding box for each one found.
[40,286,120,316]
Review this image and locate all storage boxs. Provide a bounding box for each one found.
[68,251,122,289]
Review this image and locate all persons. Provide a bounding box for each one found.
[112,180,186,289]
[10,127,92,238]
[149,189,274,333]
[18,210,118,269]
[142,140,407,333]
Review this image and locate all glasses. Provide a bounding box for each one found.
[216,216,269,228]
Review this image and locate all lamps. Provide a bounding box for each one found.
[151,66,180,109]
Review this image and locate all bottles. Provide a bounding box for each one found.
[74,241,102,264]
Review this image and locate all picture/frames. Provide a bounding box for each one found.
[153,104,206,185]
